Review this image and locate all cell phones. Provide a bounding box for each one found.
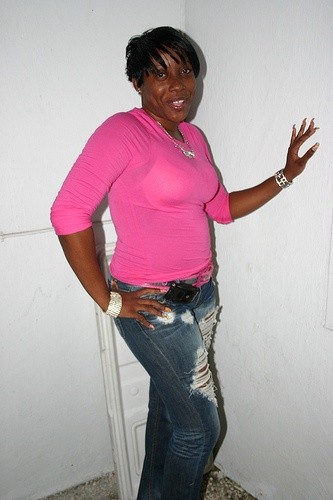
[163,284,200,304]
